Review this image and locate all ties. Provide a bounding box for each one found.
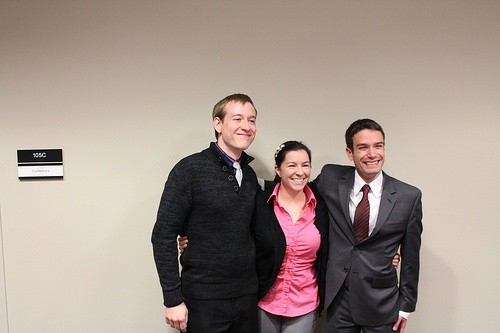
[353,184,371,244]
[231,161,243,188]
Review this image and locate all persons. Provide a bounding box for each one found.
[313,119,422,333]
[180,141,330,333]
[151,94,264,333]
